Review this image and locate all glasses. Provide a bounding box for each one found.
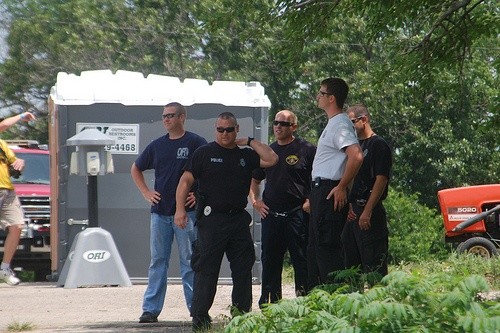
[319,91,328,97]
[352,117,362,123]
[273,120,293,126]
[216,126,237,132]
[161,113,180,118]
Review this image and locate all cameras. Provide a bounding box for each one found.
[10,166,22,179]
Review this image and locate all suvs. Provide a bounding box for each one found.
[0,139,50,283]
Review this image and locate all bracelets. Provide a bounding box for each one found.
[19,113,26,119]
[253,201,258,207]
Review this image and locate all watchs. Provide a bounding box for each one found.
[248,137,254,146]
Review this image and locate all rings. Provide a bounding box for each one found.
[364,221,369,224]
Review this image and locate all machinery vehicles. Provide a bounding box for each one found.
[437,184,500,257]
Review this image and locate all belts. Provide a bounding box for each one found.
[268,206,302,216]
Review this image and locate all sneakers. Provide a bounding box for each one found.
[0,265,20,285]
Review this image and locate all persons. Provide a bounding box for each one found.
[0,111,38,286]
[173,113,280,331]
[130,101,211,322]
[248,109,317,309]
[344,104,392,295]
[303,77,363,295]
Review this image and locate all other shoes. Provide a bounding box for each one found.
[192,318,211,332]
[139,312,158,323]
